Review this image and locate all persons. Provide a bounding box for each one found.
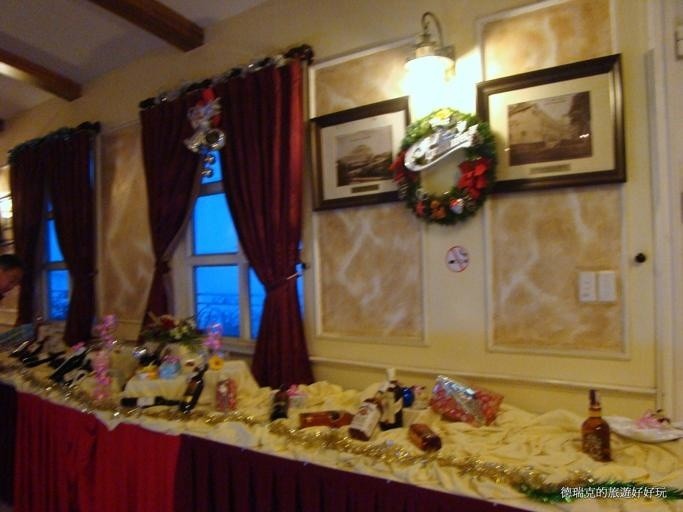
[0,253,26,302]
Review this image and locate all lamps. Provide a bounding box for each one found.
[403,12,457,81]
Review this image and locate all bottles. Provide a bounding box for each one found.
[178,367,206,414]
[348,382,388,441]
[408,422,441,455]
[299,410,355,430]
[9,335,67,368]
[49,346,93,387]
[581,388,611,465]
[270,383,290,421]
[120,396,182,408]
[380,368,404,431]
[122,341,168,391]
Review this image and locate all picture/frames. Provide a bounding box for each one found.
[475,52,626,193]
[307,95,411,212]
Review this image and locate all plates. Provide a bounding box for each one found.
[607,416,682,445]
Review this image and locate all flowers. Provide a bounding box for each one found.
[138,307,206,357]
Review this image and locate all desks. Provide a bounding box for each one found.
[0,349,683,511]
